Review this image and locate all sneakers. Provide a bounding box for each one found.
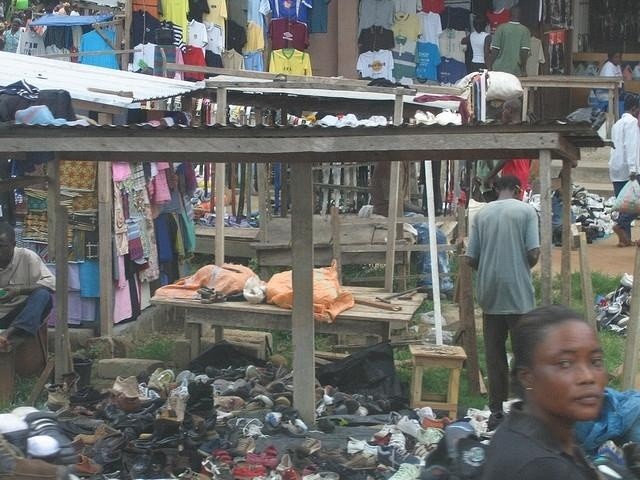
[594,273,633,334]
[570,183,637,243]
[2,365,506,479]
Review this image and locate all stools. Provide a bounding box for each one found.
[407,343,467,421]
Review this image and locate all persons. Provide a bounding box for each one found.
[0,1,83,53]
[464,12,492,74]
[587,47,623,109]
[609,93,640,246]
[483,305,607,477]
[487,97,531,202]
[464,175,539,434]
[0,222,56,352]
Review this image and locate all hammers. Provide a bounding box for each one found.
[376,287,422,304]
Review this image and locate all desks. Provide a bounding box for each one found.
[518,74,624,140]
[146,286,430,359]
